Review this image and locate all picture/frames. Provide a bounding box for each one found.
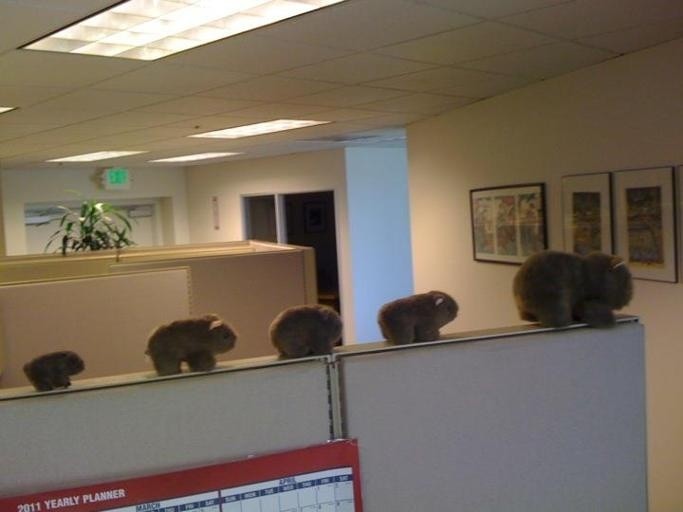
[266,200,326,234]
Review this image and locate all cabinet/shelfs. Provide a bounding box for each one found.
[467,166,678,285]
